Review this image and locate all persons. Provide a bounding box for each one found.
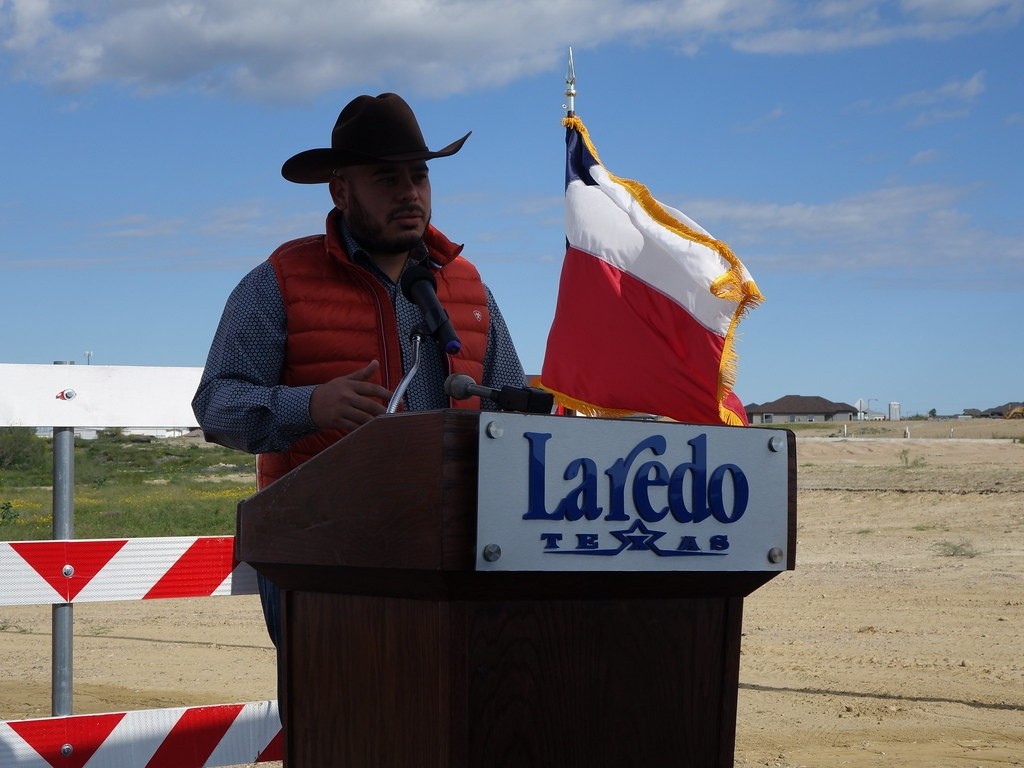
[192,92,523,643]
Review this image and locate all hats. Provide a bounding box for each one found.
[280,93,473,185]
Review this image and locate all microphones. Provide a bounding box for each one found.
[444,373,555,415]
[400,265,461,355]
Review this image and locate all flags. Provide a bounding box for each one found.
[533,119,764,428]
